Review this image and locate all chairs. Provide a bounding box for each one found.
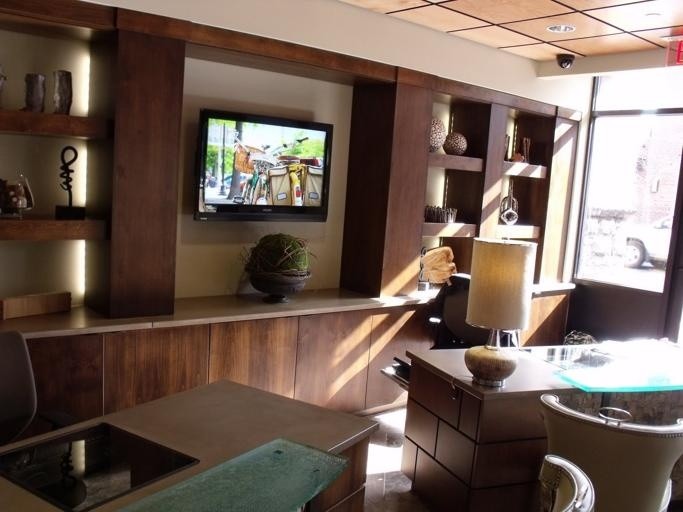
[431,273,520,349]
[538,454,596,512]
[0,330,73,447]
[538,394,683,512]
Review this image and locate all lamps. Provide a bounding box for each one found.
[465,237,538,389]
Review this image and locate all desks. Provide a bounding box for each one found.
[380,340,682,512]
[1,378,379,512]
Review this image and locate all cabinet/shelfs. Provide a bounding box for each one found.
[0,0,186,318]
[340,66,582,299]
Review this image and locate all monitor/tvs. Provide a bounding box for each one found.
[193,108,333,222]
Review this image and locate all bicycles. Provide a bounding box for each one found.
[227,134,322,204]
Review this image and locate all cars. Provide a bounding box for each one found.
[624,214,674,274]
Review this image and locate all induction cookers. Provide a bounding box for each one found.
[1,422,201,511]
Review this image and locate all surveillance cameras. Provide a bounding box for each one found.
[556,54,575,69]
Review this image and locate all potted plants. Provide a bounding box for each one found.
[238,232,312,305]
